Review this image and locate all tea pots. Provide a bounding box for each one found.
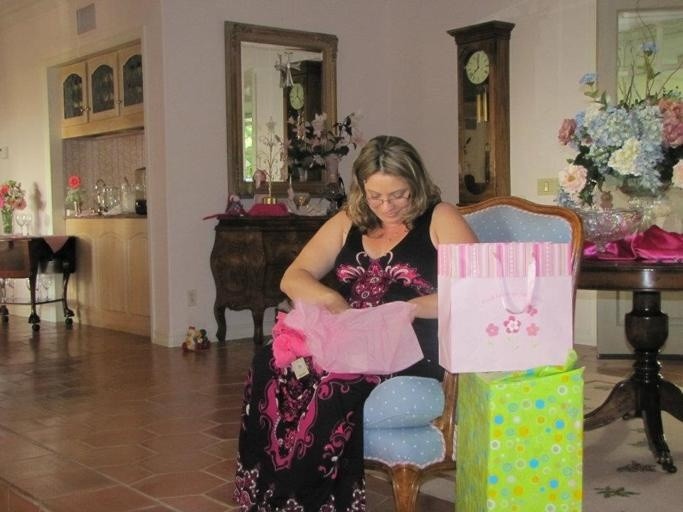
[223,18,339,200]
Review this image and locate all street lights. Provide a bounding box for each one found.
[437,241,575,371]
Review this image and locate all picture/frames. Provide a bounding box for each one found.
[575,258,682,476]
[0,233,76,326]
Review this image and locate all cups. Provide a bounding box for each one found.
[360,190,413,209]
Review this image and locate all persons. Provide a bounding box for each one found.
[232,136,480,512]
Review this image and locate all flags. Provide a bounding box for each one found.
[71,201,82,215]
[323,158,345,216]
[627,183,682,235]
[1,209,13,233]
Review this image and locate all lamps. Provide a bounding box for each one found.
[357,194,583,510]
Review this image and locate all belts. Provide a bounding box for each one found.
[15,214,33,237]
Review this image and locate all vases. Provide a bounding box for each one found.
[64,173,87,205]
[276,112,326,177]
[0,177,27,233]
[302,107,361,173]
[554,37,683,206]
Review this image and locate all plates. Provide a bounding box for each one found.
[209,216,330,345]
[66,219,148,329]
[116,43,143,116]
[57,50,118,129]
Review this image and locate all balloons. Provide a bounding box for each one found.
[281,59,322,181]
[444,19,515,207]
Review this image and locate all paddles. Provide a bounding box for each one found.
[369,368,681,512]
